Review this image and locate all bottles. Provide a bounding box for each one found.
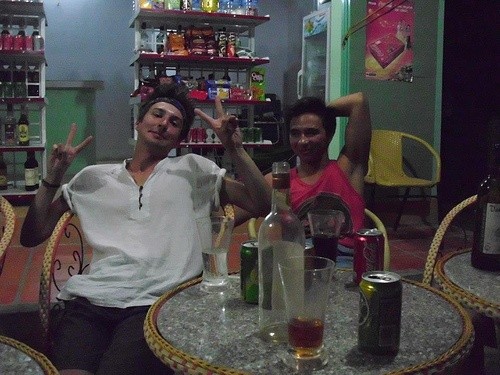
[0,101,17,146]
[31,149,39,189]
[139,61,266,102]
[0,150,7,190]
[24,149,35,191]
[0,64,39,98]
[257,163,306,344]
[471,143,500,272]
[138,0,259,57]
[18,105,30,146]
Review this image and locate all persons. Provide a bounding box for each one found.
[20,82,272,375]
[213,91,373,254]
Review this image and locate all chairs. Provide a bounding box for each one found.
[38,191,234,334]
[0,197,17,277]
[248,205,390,276]
[364,129,441,231]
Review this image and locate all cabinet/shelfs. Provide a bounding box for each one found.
[127,0,274,148]
[0,0,48,196]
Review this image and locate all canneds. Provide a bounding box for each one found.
[185,127,222,144]
[239,240,272,305]
[358,270,404,356]
[218,31,236,57]
[241,127,263,144]
[352,228,385,286]
[302,242,314,294]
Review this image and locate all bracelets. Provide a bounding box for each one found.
[41,180,61,188]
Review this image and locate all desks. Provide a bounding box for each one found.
[143,266,475,375]
[432,247,500,320]
[0,336,60,375]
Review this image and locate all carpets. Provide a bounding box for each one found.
[0,270,500,375]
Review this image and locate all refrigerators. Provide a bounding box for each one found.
[296,0,444,196]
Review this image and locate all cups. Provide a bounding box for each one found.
[277,255,335,371]
[194,215,235,293]
[307,210,344,284]
